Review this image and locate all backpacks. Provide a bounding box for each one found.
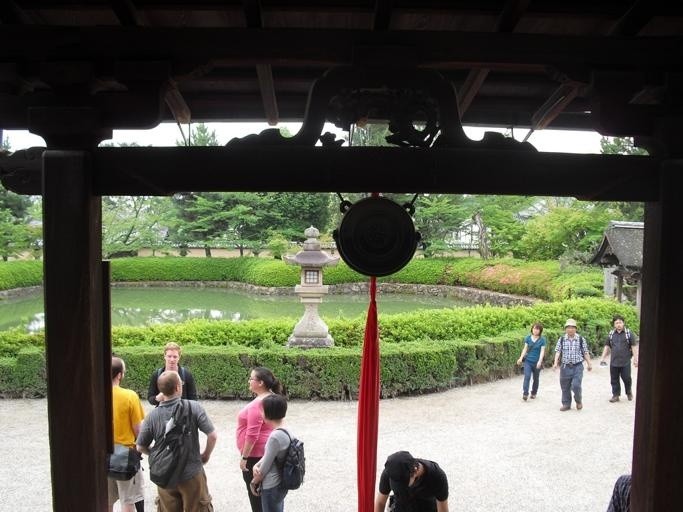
[272,428,305,490]
[149,399,189,488]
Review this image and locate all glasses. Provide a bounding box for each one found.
[249,377,260,381]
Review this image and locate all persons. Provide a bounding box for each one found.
[552,319,592,411]
[599,314,638,403]
[136,370,218,512]
[374,451,449,512]
[108,356,147,512]
[236,367,282,512]
[607,474,632,512]
[250,394,295,512]
[516,324,547,400]
[147,341,197,405]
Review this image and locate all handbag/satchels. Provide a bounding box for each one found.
[107,442,141,481]
[522,336,541,362]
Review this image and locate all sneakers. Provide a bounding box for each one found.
[530,394,536,399]
[577,402,582,409]
[523,395,528,400]
[560,405,571,411]
[609,394,619,402]
[627,393,633,400]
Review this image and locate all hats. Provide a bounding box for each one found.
[385,450,413,497]
[565,318,576,327]
